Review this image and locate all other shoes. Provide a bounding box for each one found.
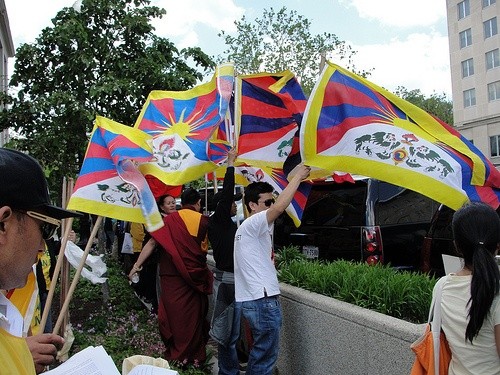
[239,363,248,370]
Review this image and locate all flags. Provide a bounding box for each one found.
[229,70,308,227]
[299,59,500,211]
[130,63,233,187]
[65,115,165,232]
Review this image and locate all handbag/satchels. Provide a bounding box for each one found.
[121,232,134,254]
[409,276,452,375]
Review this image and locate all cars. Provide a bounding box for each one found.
[273,172,442,274]
[198,185,248,226]
[418,200,500,280]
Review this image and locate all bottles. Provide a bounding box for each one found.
[132,272,139,283]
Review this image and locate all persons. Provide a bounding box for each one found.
[0,149,65,375]
[432,201,500,375]
[106,147,310,375]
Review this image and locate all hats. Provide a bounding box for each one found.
[215,190,243,208]
[181,188,201,204]
[0,148,84,221]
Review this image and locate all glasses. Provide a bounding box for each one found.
[10,207,62,240]
[257,198,276,207]
